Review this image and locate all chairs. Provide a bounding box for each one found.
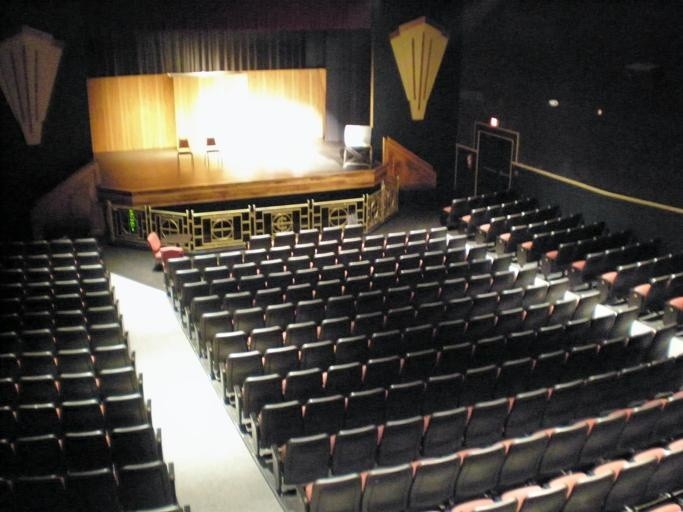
[174,135,224,172]
[339,124,374,171]
